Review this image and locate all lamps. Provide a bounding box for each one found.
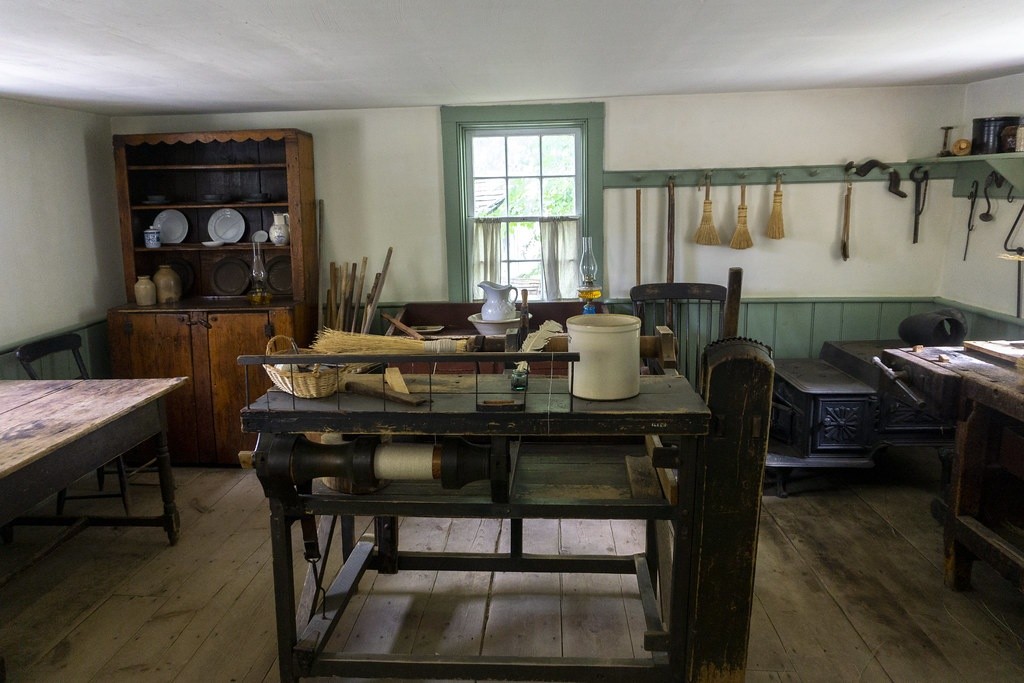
[576,236,602,314]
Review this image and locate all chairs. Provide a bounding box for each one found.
[16,333,159,515]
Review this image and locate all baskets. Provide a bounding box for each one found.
[261,336,349,398]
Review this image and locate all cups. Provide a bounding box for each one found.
[143,226,161,249]
[565,314,640,400]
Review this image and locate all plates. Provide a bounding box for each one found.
[202,241,224,248]
[153,209,189,243]
[142,200,170,205]
[199,199,225,204]
[265,255,292,295]
[409,325,444,331]
[245,198,271,202]
[207,208,245,243]
[210,256,249,295]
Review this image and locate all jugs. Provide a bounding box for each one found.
[477,280,518,320]
[268,213,291,247]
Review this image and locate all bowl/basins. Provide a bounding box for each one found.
[148,194,166,200]
[248,192,272,198]
[468,310,532,337]
[970,116,1020,155]
[200,193,223,200]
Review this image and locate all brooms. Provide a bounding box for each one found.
[728,186,753,250]
[693,174,722,246]
[767,174,785,239]
[307,325,468,369]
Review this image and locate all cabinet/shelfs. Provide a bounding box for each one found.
[107,128,318,469]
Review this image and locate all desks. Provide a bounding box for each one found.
[0,377,189,586]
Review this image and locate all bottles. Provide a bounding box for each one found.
[153,264,181,303]
[952,138,971,156]
[247,254,272,305]
[1015,117,1024,151]
[511,370,527,390]
[134,275,156,306]
